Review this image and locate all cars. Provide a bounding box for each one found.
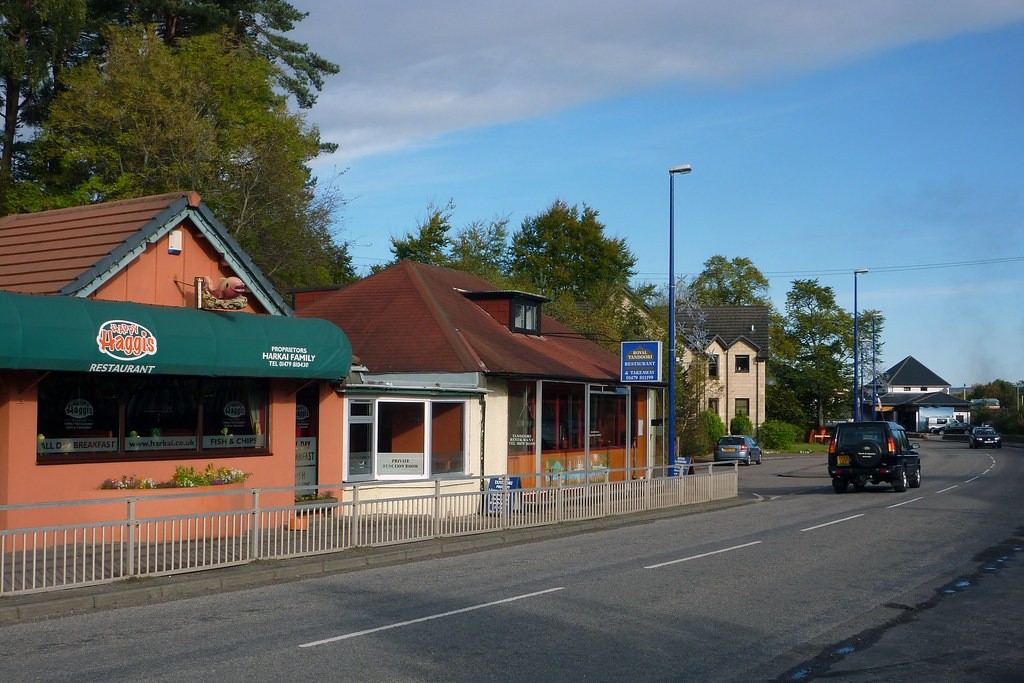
[933,422,971,435]
[969,426,1002,449]
[713,433,763,466]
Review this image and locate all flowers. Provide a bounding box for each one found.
[296,490,334,500]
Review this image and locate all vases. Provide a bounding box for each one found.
[295,497,338,504]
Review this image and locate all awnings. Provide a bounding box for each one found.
[0,290,352,380]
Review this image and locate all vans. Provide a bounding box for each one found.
[925,417,962,433]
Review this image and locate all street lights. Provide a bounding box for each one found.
[851,267,871,421]
[665,165,694,478]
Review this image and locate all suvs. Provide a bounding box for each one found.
[826,420,923,493]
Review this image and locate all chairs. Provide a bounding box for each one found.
[844,432,864,443]
